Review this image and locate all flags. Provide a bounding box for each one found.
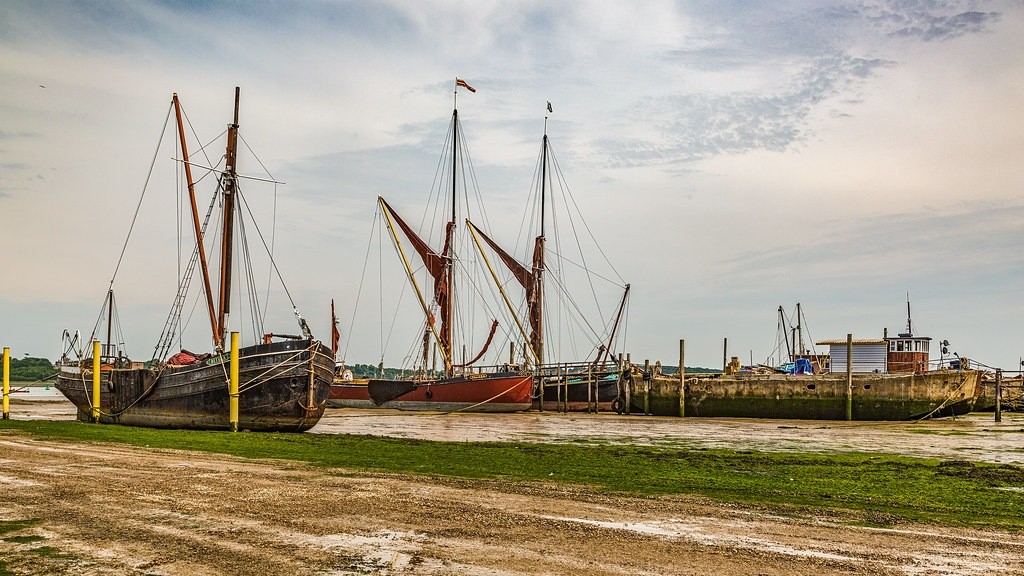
[331,302,340,353]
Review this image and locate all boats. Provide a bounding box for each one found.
[50,83,338,432]
[614,345,988,420]
[522,114,632,410]
[323,80,535,414]
